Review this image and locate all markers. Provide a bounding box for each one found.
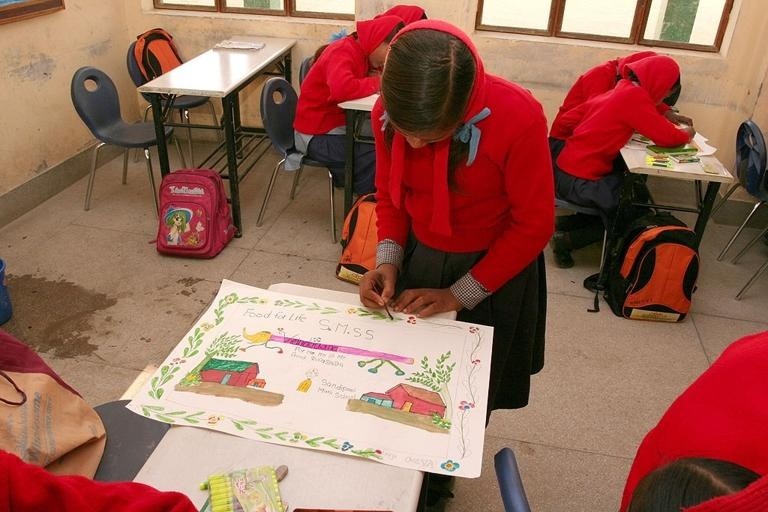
[201,466,284,512]
[646,155,672,168]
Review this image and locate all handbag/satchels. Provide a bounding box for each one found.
[1,327,105,482]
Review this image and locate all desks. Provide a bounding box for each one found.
[338,71,383,218]
[105,280,458,511]
[137,34,297,238]
[597,107,733,290]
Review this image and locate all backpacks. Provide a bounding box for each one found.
[132,27,184,123]
[336,193,377,287]
[584,211,700,323]
[148,169,238,259]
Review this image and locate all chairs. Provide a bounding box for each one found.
[127,39,222,170]
[0,329,170,482]
[298,56,315,89]
[256,77,349,244]
[710,120,767,261]
[554,197,607,271]
[71,67,187,220]
[496,445,531,512]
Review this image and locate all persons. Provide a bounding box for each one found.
[625,453,762,512]
[374,4,428,24]
[549,55,696,268]
[358,20,556,511]
[547,50,693,164]
[293,14,404,203]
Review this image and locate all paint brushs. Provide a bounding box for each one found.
[370,280,394,321]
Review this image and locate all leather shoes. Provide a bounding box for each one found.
[425,478,455,512]
[546,214,574,269]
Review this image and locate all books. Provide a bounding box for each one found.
[669,154,700,163]
